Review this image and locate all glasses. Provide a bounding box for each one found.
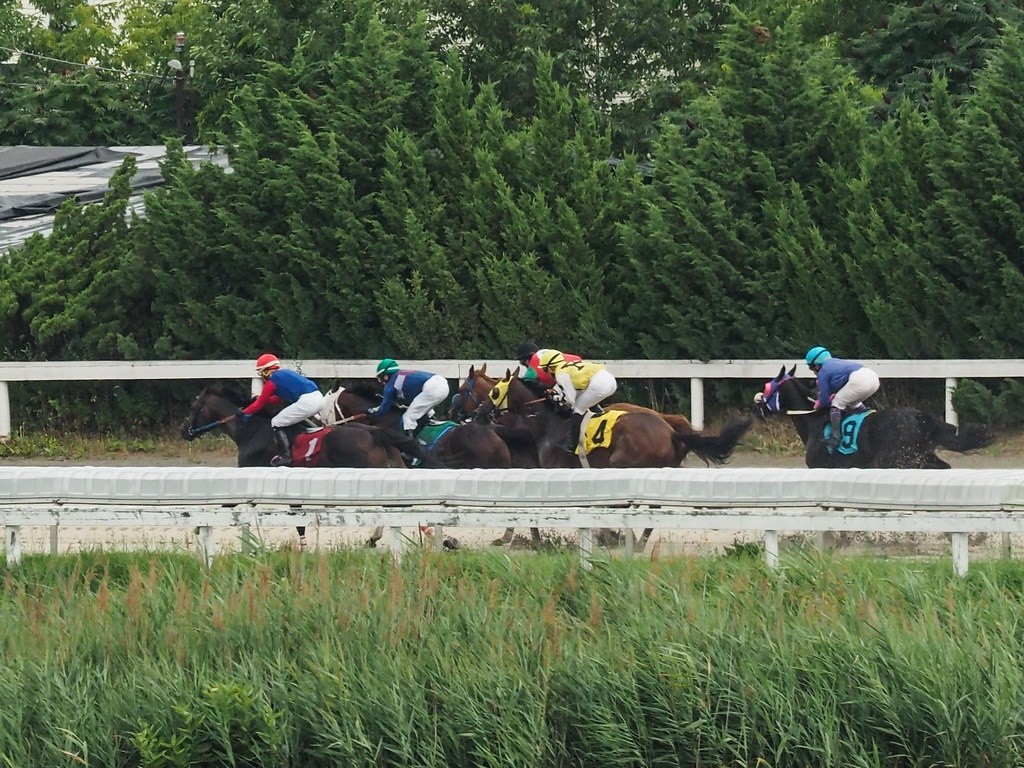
[378,376,385,384]
[809,364,815,370]
[521,357,527,364]
[543,367,549,373]
[258,371,263,376]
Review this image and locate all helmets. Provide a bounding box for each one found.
[376,359,400,376]
[256,354,279,372]
[806,347,830,365]
[537,350,564,369]
[516,343,541,358]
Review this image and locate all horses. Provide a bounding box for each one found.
[474,366,751,468]
[179,381,439,469]
[752,363,997,469]
[448,362,720,467]
[304,378,541,469]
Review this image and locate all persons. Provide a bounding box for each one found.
[519,342,582,396]
[806,347,879,445]
[376,359,450,461]
[242,354,324,465]
[539,349,618,455]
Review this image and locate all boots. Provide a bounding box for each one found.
[398,429,415,461]
[270,428,293,466]
[820,407,845,447]
[556,410,589,455]
